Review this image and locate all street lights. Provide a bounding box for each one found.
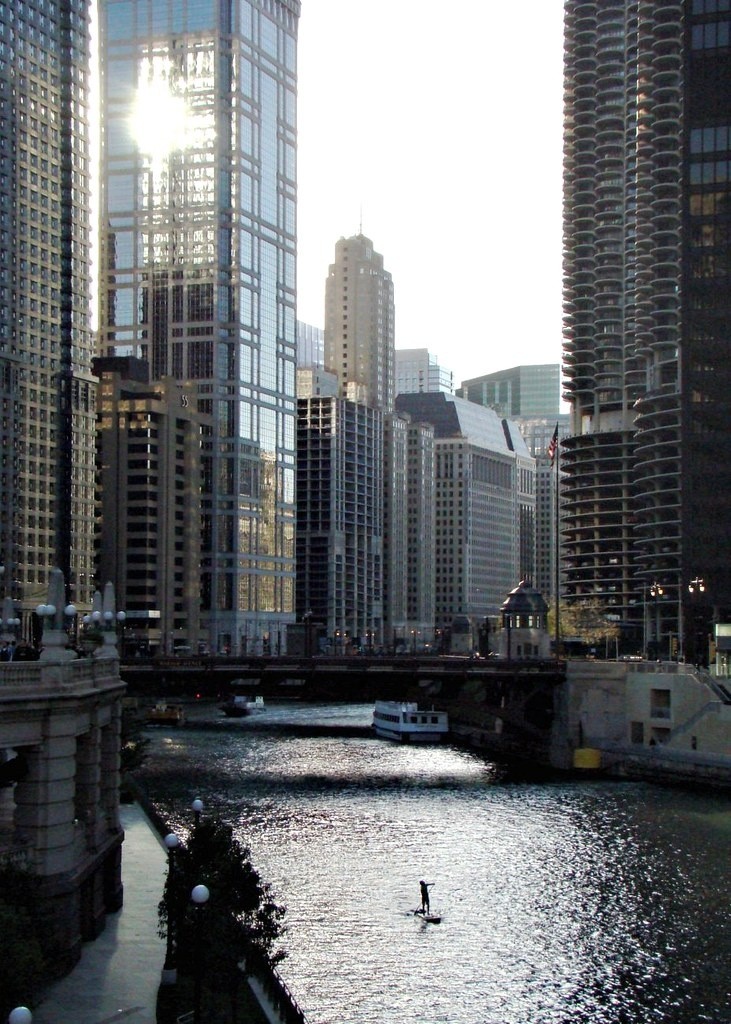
[192,796,204,882]
[688,577,704,671]
[191,882,209,1024]
[411,630,421,657]
[303,612,308,657]
[365,632,375,655]
[649,580,664,661]
[434,626,446,655]
[307,610,314,658]
[337,629,346,656]
[162,833,180,970]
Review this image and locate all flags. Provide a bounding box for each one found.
[547,421,558,470]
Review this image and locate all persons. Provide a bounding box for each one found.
[419,880,434,916]
[0,639,30,662]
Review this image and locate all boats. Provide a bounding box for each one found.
[373,699,450,744]
[145,702,185,726]
[218,694,266,717]
